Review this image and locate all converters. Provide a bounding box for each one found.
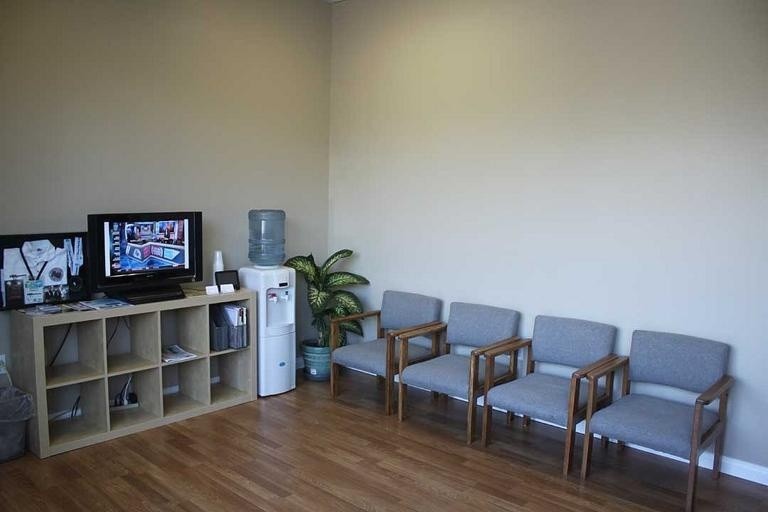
[128,392,137,403]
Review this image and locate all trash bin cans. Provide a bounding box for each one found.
[0,386,32,464]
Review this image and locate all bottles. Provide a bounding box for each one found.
[212,250,224,284]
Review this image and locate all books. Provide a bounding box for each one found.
[63,302,94,312]
[79,296,131,309]
[225,305,248,348]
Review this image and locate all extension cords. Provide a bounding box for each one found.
[110,401,139,412]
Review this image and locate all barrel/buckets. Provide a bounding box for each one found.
[247,209,286,267]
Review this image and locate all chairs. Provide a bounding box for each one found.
[392,299,525,447]
[580,326,740,510]
[327,287,444,417]
[477,309,620,479]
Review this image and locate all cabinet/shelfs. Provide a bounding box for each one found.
[0,277,260,459]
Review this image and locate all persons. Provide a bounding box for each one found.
[134,228,141,240]
[164,228,171,238]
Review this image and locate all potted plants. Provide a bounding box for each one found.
[286,245,373,384]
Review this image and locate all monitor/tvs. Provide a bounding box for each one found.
[0,232,92,312]
[87,211,203,305]
